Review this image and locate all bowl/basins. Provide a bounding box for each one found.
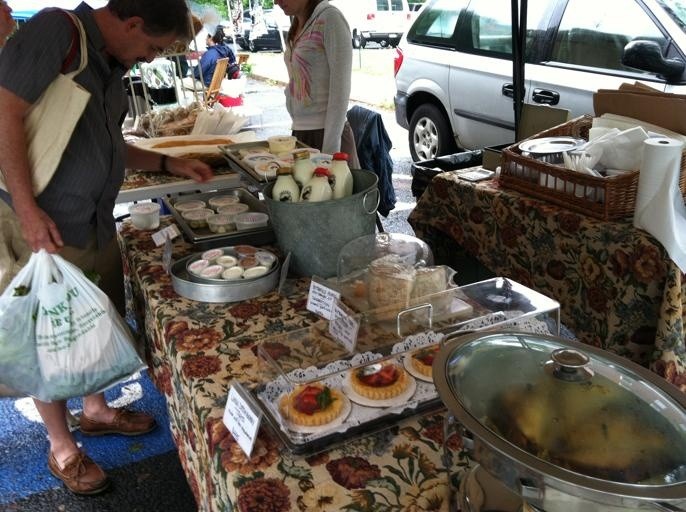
[243,133,334,177]
[175,195,272,233]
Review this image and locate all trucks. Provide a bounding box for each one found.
[10,9,37,30]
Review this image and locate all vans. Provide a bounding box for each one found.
[327,0,424,47]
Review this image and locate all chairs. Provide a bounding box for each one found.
[238,52,250,65]
[202,54,230,106]
[345,105,397,233]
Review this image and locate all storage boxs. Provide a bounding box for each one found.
[481,142,517,172]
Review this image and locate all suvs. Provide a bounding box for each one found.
[391,0,686,166]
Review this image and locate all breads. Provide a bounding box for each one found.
[367,252,447,316]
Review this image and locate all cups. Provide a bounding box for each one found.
[127,203,161,232]
[189,245,276,277]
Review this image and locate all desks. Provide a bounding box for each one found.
[409,166,686,396]
[113,166,242,206]
[117,206,585,510]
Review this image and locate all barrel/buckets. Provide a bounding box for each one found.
[239,54,249,68]
[262,162,380,279]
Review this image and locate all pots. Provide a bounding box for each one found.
[433,326,686,511]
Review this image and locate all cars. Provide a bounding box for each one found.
[223,7,292,52]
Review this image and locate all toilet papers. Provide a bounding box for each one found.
[633,137,686,275]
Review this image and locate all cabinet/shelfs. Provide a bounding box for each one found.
[116,1,207,135]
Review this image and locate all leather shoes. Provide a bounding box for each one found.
[47,445,112,495]
[79,406,159,436]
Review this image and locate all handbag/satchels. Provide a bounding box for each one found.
[1,60,90,200]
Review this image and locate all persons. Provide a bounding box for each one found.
[270,0,365,173]
[0,2,192,497]
[0,0,21,46]
[192,24,237,88]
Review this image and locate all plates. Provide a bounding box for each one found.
[342,342,443,407]
[519,133,586,156]
[274,387,351,430]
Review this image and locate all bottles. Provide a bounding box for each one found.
[271,149,356,201]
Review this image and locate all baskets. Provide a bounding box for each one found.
[499,116,684,222]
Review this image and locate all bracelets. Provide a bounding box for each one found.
[158,153,168,174]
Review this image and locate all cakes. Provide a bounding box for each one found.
[279,343,439,425]
